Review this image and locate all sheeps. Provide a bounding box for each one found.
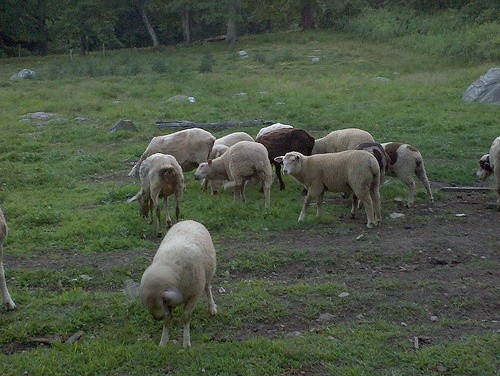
[476,136,499,205]
[124,122,434,239]
[139,219,218,350]
[0,207,18,311]
